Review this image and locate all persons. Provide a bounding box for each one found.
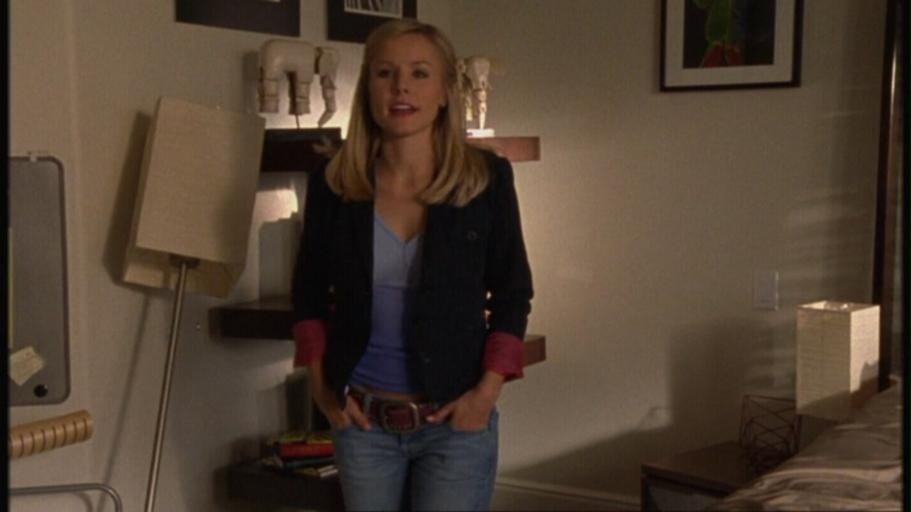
[291,20,536,512]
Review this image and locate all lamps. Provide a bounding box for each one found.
[122,96,266,512]
[796,299,881,424]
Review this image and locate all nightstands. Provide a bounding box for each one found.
[640,441,788,512]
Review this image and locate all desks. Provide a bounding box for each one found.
[227,458,412,511]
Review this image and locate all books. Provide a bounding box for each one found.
[267,419,340,478]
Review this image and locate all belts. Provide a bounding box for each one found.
[346,389,448,436]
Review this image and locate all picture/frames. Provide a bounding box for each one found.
[660,0,804,92]
[176,0,300,38]
[328,0,417,43]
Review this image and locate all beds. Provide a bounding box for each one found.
[707,383,903,510]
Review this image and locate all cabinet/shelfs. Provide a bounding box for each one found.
[210,137,546,366]
